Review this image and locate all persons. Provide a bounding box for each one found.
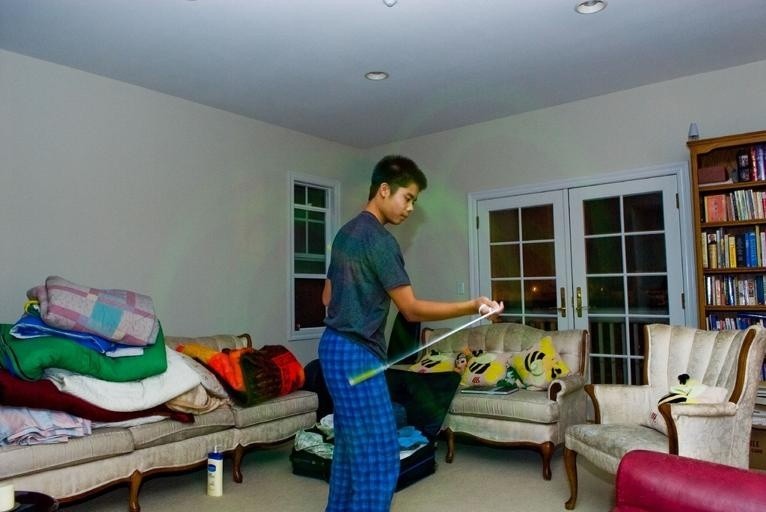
[317,155,505,511]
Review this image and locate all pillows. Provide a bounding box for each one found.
[639,371,730,436]
[508,334,570,391]
[457,349,511,387]
[406,350,468,373]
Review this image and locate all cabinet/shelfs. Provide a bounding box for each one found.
[686,129,766,429]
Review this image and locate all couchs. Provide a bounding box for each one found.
[407,322,600,481]
[0,333,318,511]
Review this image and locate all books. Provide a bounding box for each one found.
[700,148,766,426]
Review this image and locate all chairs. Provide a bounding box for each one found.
[610,449,765,511]
[565,322,766,512]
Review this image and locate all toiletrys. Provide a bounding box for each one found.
[207,445,224,497]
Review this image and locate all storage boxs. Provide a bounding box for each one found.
[749,431,766,470]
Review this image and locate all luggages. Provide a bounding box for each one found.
[289,368,461,494]
